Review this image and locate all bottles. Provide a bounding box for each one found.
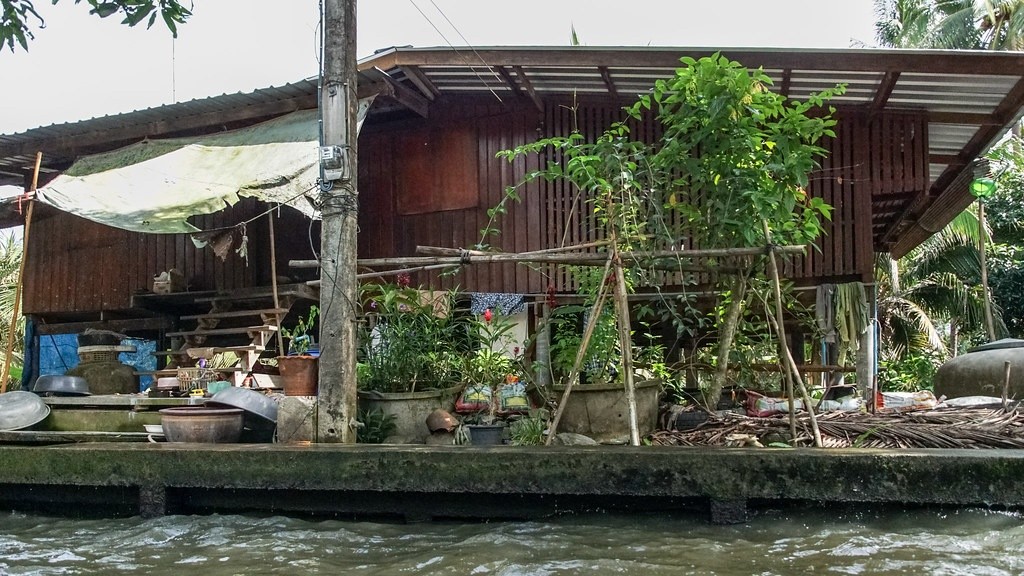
[199,356,205,368]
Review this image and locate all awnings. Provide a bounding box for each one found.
[33,92,412,265]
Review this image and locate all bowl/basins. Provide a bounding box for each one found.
[0,391,51,430]
[159,407,244,443]
[30,374,92,394]
[143,425,163,433]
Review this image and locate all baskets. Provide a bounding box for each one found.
[80,352,120,363]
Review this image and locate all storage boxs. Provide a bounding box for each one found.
[153,271,188,294]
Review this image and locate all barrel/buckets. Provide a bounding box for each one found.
[167,349,171,366]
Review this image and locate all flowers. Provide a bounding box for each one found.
[353,264,671,426]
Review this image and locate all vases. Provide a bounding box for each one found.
[160,407,245,443]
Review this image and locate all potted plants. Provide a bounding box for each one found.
[467,426,505,445]
[357,380,465,441]
[274,305,320,396]
[552,377,663,444]
